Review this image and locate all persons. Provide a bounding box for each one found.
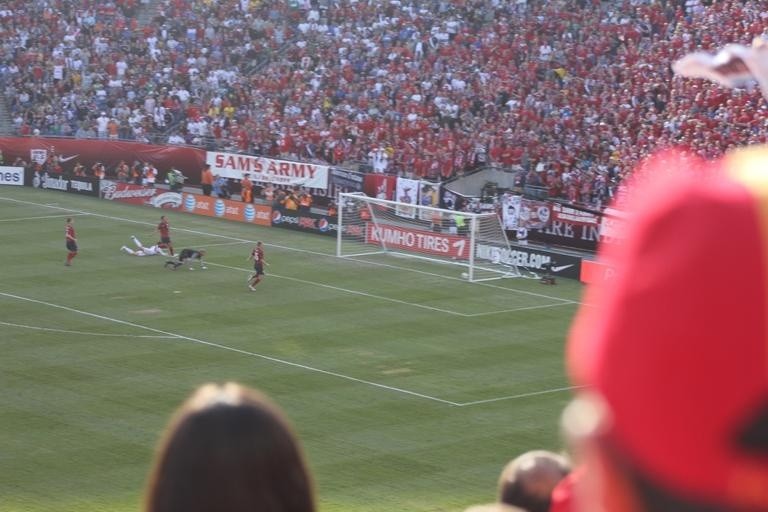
[497,449,576,511]
[0,0,767,292]
[147,384,315,512]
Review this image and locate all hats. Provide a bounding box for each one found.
[551,461,647,512]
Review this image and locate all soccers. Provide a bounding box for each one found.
[459,272,469,280]
